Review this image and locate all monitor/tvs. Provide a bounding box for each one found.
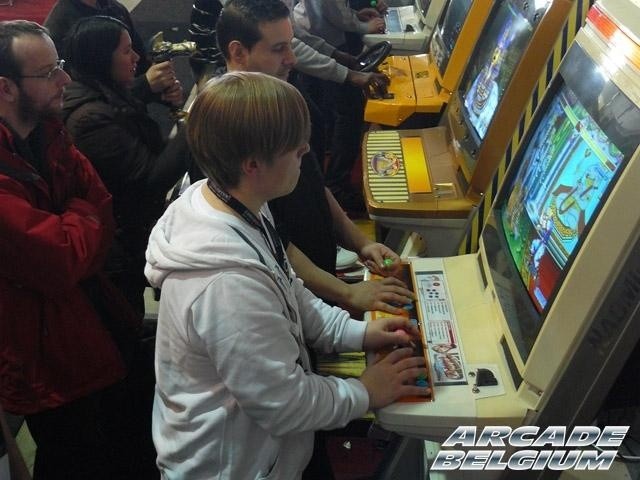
[501,82,625,315]
[462,0,533,140]
[440,0,470,47]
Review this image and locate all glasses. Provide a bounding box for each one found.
[19,61,64,80]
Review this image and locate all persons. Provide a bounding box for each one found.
[188,0,228,86]
[57,16,186,273]
[43,0,145,63]
[137,72,433,479]
[0,21,149,480]
[218,0,416,315]
[281,0,392,221]
